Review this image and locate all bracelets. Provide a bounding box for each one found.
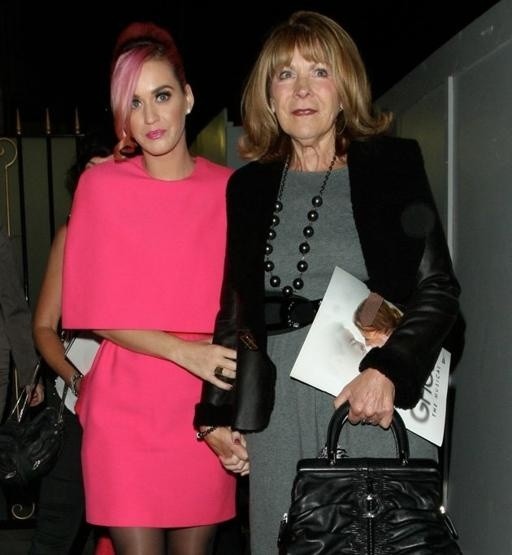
[194,424,218,440]
[69,374,82,398]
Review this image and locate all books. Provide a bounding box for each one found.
[289,265,453,449]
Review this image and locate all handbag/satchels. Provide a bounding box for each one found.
[0,403,67,485]
[276,399,467,554]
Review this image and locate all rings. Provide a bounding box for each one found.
[360,416,372,426]
[213,365,223,377]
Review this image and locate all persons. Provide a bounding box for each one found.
[60,19,235,554]
[352,295,403,349]
[193,9,463,555]
[0,234,46,430]
[31,140,142,554]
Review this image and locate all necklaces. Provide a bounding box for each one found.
[263,150,340,297]
